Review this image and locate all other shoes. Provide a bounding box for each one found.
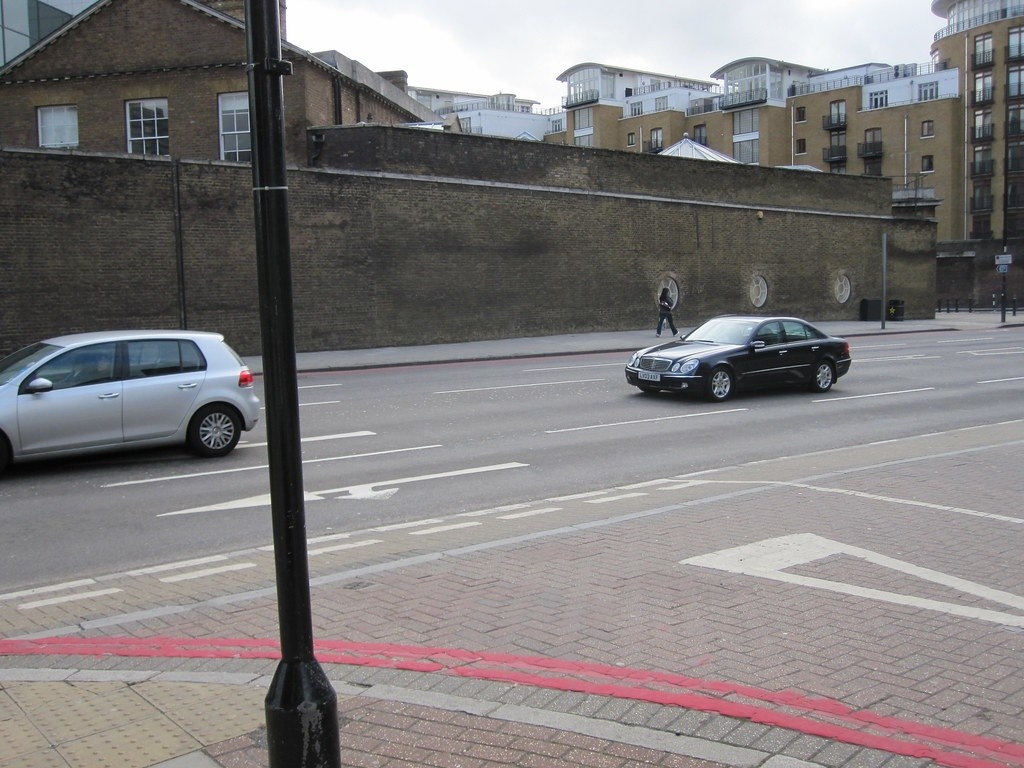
[673,331,679,337]
[656,333,662,337]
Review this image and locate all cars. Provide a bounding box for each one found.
[0,330,261,472]
[623,316,852,404]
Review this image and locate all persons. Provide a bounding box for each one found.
[656,288,679,337]
[51,351,112,389]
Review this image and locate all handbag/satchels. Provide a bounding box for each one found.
[660,301,670,310]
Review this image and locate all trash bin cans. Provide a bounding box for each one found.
[861,298,882,321]
[889,299,905,321]
